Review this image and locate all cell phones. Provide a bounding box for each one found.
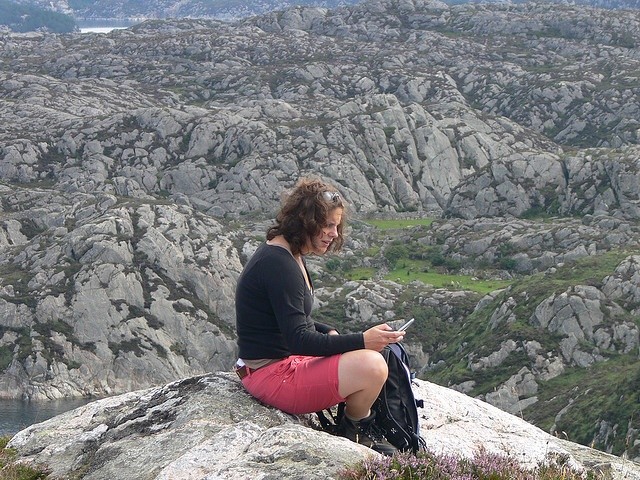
[386,318,417,331]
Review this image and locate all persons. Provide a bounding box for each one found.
[234,177,407,456]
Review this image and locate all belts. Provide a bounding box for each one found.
[234,359,281,379]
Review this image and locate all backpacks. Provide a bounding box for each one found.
[316,342,427,455]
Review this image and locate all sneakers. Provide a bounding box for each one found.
[338,407,398,457]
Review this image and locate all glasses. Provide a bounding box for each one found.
[323,191,340,201]
